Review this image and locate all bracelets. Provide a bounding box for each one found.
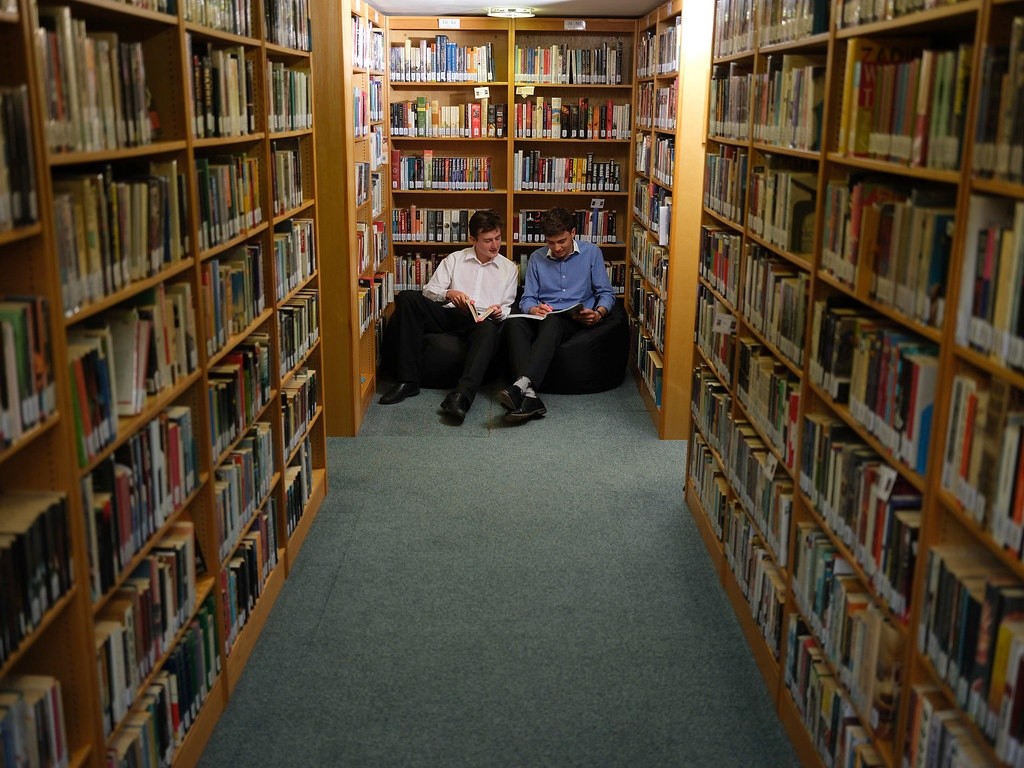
[597,309,603,318]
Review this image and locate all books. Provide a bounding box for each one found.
[0,0,1024,768]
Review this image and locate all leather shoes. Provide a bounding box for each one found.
[504,396,547,422]
[440,393,467,421]
[379,382,420,405]
[501,385,525,411]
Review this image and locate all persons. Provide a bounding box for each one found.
[498,206,616,421]
[377,211,518,421]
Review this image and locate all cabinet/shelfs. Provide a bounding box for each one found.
[0,0,1024,768]
